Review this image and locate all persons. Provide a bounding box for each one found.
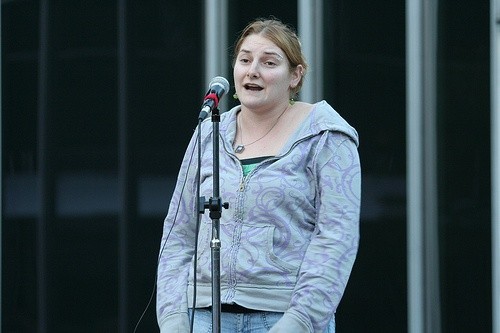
[156,18,362,333]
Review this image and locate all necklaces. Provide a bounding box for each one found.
[233,102,291,153]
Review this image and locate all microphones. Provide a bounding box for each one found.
[198,76,230,122]
